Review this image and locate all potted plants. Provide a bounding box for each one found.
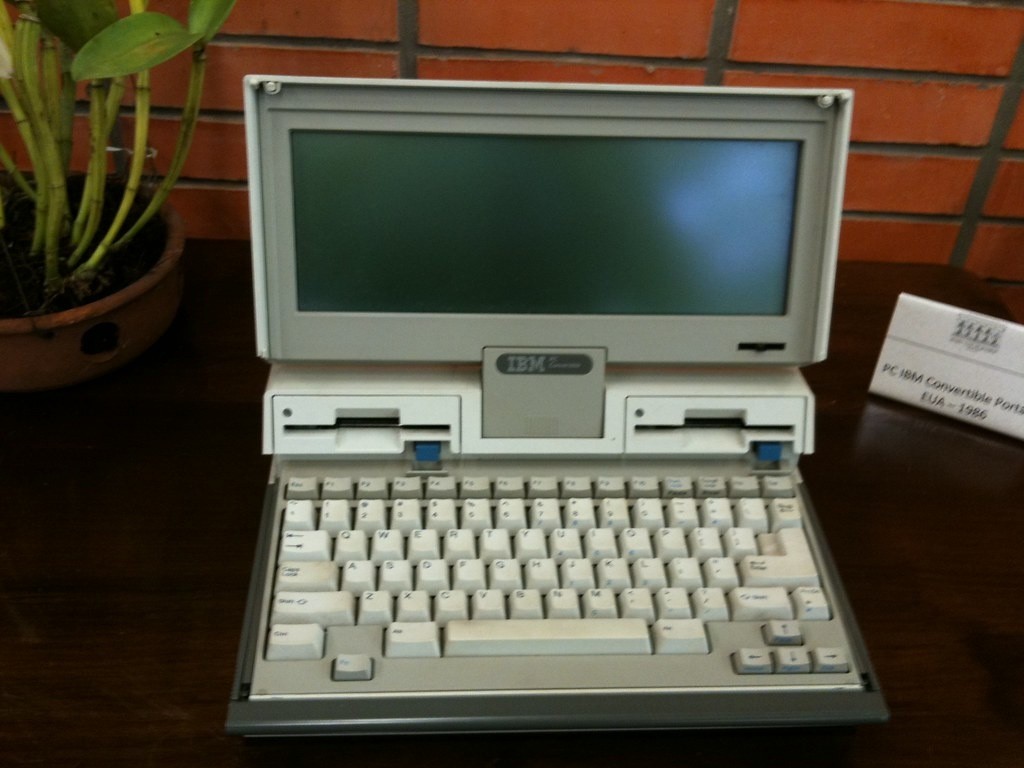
[1,1,239,395]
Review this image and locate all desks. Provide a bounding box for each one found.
[0,236,1024,768]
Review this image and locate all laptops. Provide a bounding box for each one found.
[223,73,891,737]
